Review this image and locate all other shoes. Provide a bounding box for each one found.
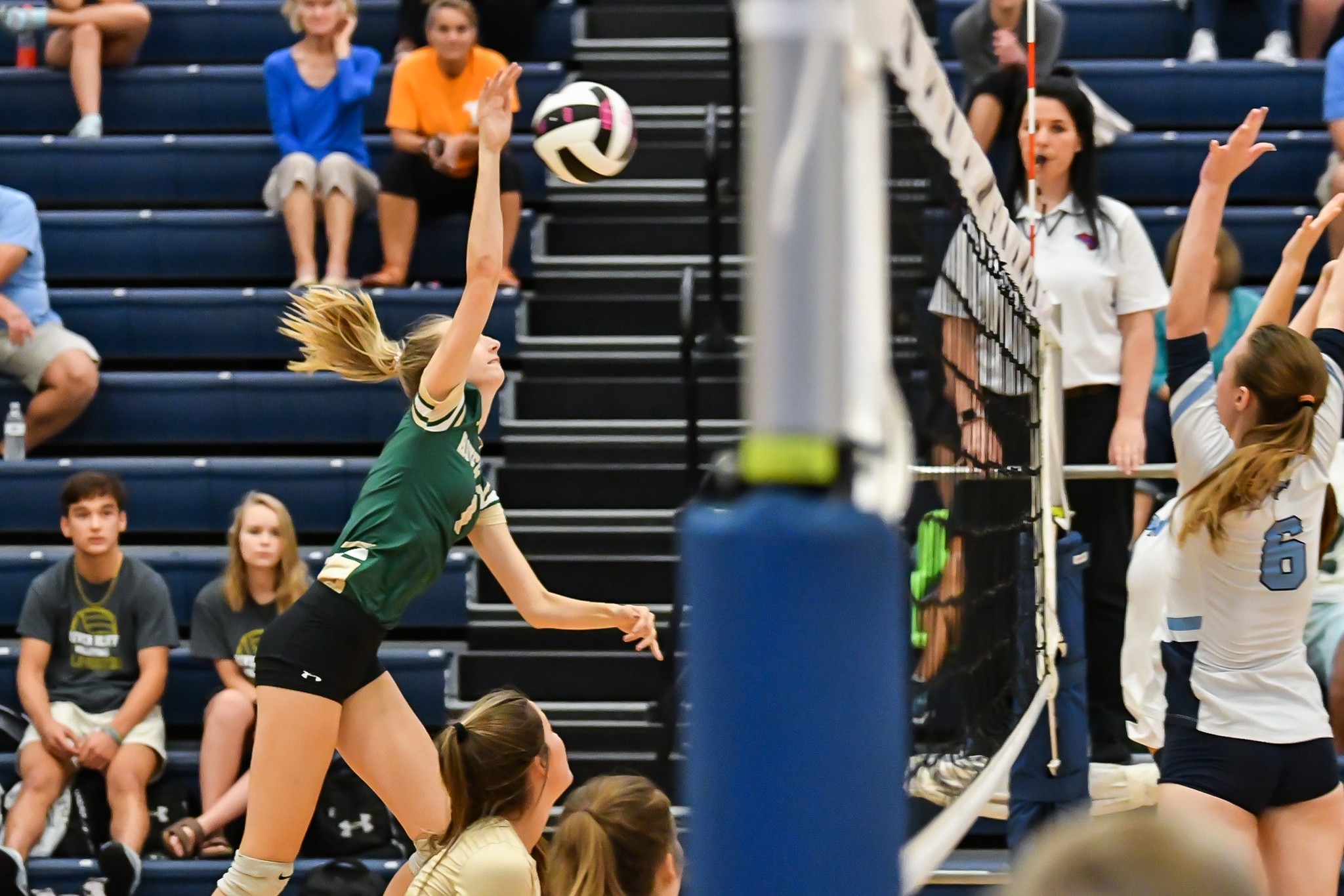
[320,277,348,289]
[5,5,48,33]
[290,278,320,288]
[498,267,520,290]
[76,112,104,140]
[360,273,408,289]
[1252,30,1294,61]
[98,841,144,896]
[0,844,30,896]
[1187,28,1221,66]
[909,678,931,726]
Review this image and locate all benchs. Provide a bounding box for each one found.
[2,0,1343,896]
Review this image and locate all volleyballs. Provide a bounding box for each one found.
[529,81,638,184]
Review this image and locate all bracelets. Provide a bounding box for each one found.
[103,722,124,746]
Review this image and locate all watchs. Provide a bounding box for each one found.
[958,409,986,431]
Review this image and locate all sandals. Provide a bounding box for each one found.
[162,816,206,859]
[198,834,235,860]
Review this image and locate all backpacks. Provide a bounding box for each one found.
[298,755,409,862]
[2,779,188,858]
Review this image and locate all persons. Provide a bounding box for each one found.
[904,1,1344,896]
[540,776,684,896]
[0,0,150,138]
[0,471,181,896]
[1,184,100,457]
[361,0,521,288]
[403,690,573,896]
[163,492,313,859]
[209,63,664,896]
[263,0,381,288]
